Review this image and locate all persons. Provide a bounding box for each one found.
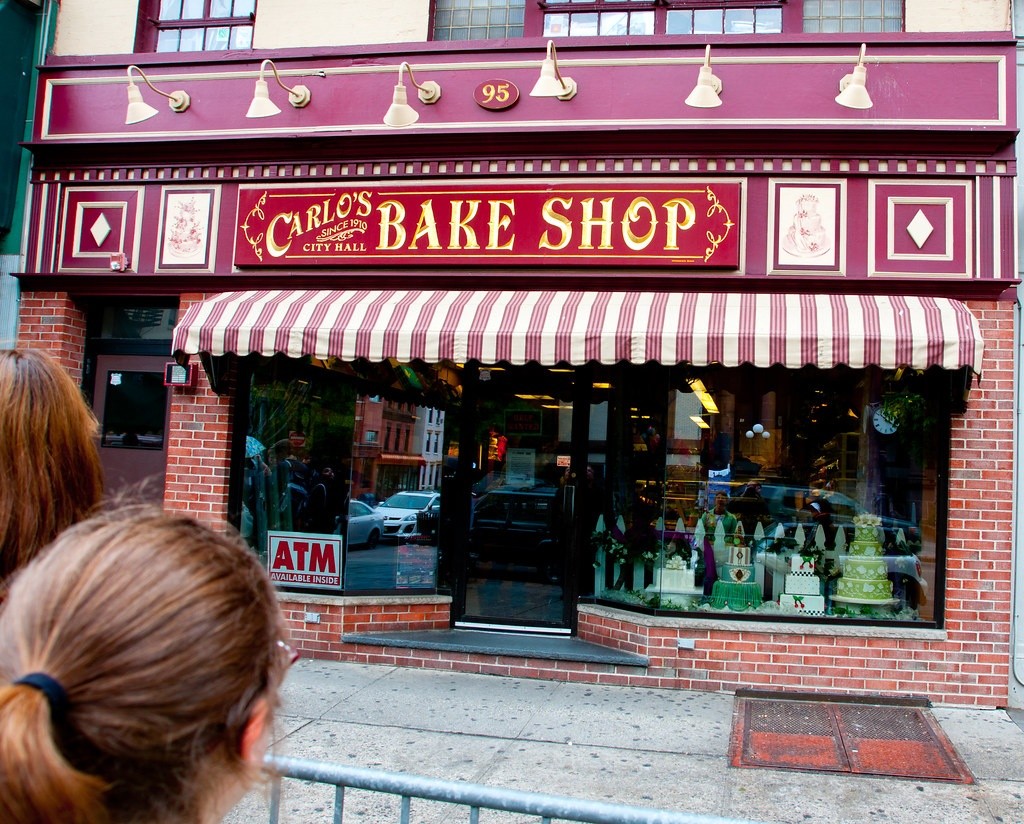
[727,482,770,545]
[245,436,335,533]
[0,346,106,607]
[701,491,737,605]
[0,504,291,824]
[800,489,839,550]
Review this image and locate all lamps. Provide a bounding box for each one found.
[835,43,873,108]
[529,40,578,101]
[383,61,442,128]
[125,65,191,125]
[246,59,311,118]
[685,45,723,108]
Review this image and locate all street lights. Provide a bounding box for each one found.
[745,424,771,456]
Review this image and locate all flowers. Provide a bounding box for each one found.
[666,554,686,569]
[853,512,883,528]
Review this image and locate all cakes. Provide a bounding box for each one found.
[777,552,825,612]
[720,534,755,582]
[655,556,695,592]
[836,513,893,600]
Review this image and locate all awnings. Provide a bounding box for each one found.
[171,290,981,410]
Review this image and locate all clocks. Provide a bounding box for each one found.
[870,406,901,437]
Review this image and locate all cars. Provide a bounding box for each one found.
[225,502,255,545]
[374,491,440,540]
[472,486,559,578]
[759,484,922,551]
[748,521,928,620]
[334,500,384,551]
